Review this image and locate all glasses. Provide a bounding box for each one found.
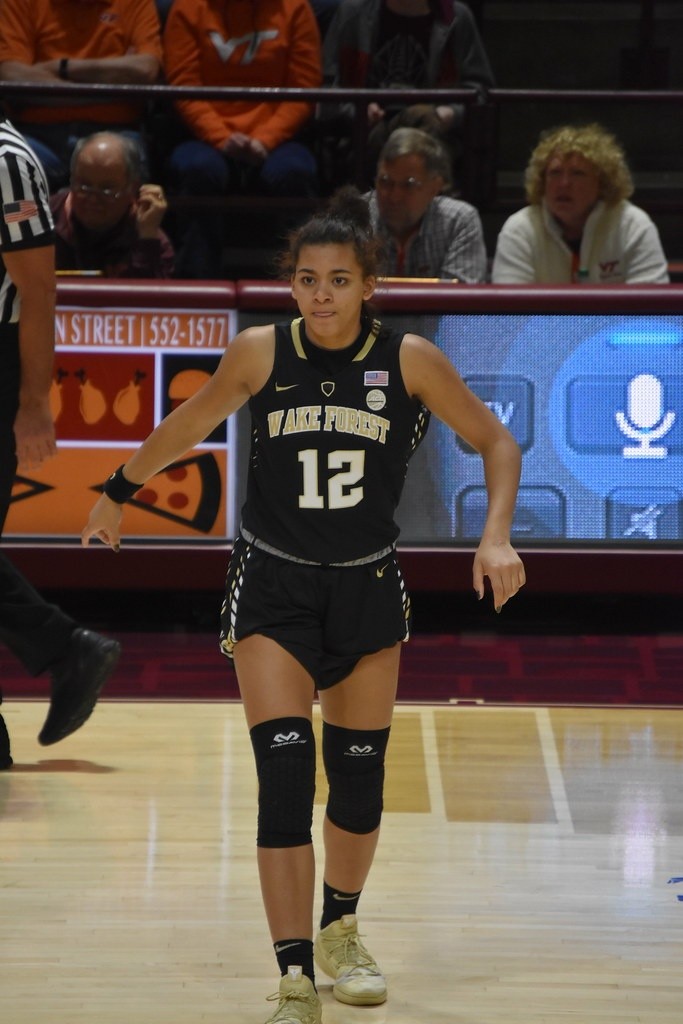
[376,174,435,199]
[73,179,132,202]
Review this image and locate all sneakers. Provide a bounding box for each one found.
[312,914,387,1004]
[264,965,322,1024]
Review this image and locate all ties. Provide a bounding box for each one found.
[396,240,408,278]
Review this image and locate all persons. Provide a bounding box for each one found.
[492,125,670,287]
[0,107,126,769]
[0,1,503,282]
[82,190,528,1024]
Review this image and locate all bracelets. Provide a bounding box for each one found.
[103,464,143,505]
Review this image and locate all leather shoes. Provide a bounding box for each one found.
[37,627,121,745]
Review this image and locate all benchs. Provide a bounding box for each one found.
[161,196,324,280]
